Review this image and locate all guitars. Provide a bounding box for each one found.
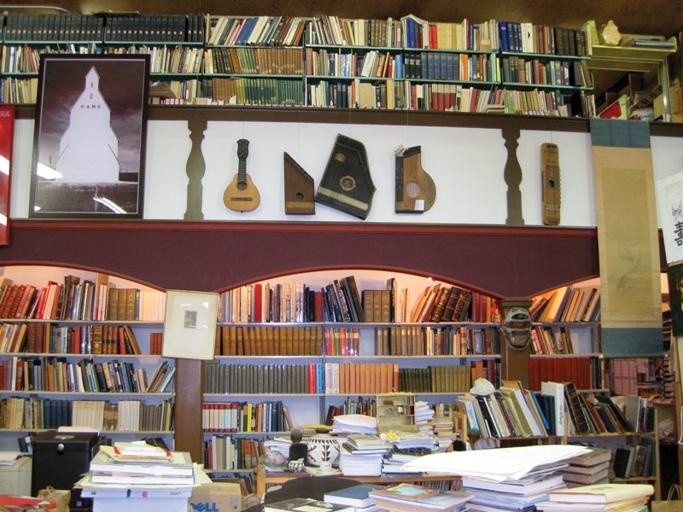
[223,139,259,211]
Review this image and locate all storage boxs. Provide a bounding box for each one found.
[1,431,253,510]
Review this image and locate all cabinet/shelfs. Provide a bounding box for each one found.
[202,263,518,511]
[517,269,683,510]
[1,262,176,448]
[2,1,683,126]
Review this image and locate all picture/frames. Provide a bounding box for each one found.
[27,50,153,221]
[160,288,220,362]
